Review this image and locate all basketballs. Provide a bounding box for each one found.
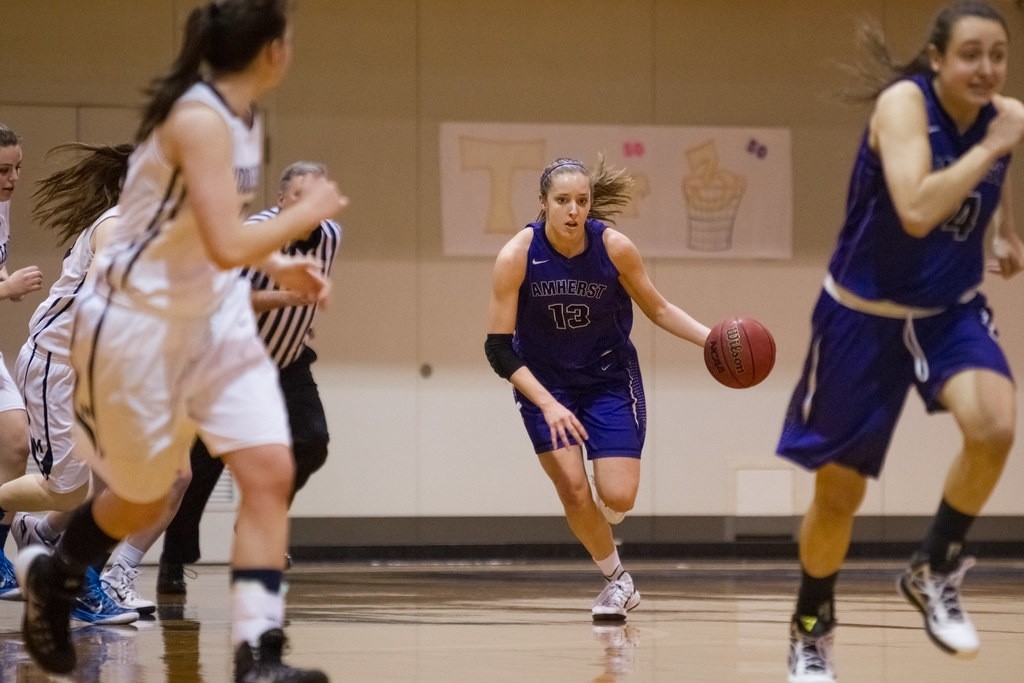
[704,315,776,389]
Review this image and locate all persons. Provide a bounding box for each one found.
[15,0,350,683]
[483,158,713,621]
[775,3,1024,683]
[0,125,341,619]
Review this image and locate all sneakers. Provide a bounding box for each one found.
[232,629,330,683]
[11,512,59,555]
[591,570,641,621]
[593,477,627,526]
[156,554,199,594]
[788,619,838,683]
[0,550,24,601]
[16,545,77,673]
[896,549,982,661]
[73,572,141,625]
[100,565,157,616]
